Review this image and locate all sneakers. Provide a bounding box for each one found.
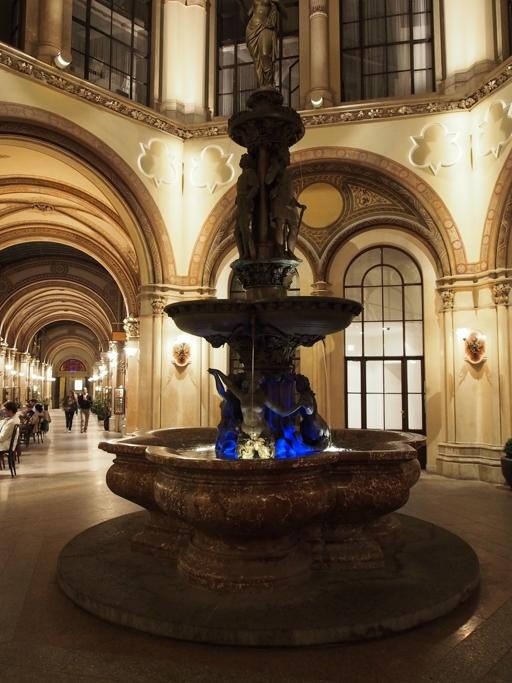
[64,427,89,434]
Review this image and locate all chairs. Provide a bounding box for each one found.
[0,415,44,478]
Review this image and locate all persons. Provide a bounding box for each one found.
[19,398,51,439]
[62,389,78,432]
[244,0,290,88]
[78,387,94,434]
[207,367,333,461]
[0,401,21,455]
[232,140,307,264]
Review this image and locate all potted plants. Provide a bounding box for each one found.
[92,399,111,431]
